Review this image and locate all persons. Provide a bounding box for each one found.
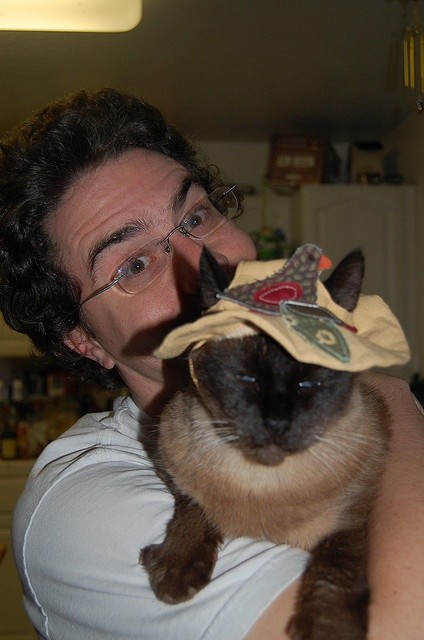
[0,87,422,634]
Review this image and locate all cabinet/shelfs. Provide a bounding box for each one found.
[293,184,419,379]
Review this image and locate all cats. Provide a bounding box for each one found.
[135,244,393,640]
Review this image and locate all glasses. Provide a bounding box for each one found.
[76,185,239,306]
